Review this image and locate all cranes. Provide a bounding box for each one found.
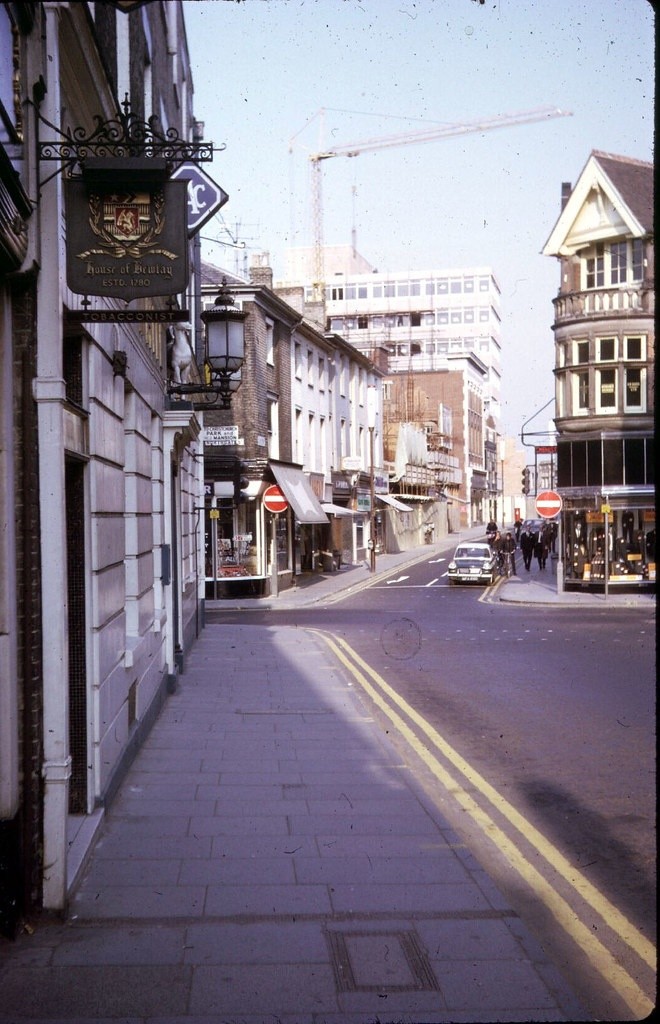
[287,107,573,302]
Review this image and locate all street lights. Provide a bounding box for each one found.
[368,386,377,575]
[499,440,506,529]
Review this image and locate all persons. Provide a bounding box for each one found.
[514,518,523,542]
[521,526,535,571]
[497,532,517,576]
[534,526,549,570]
[548,522,557,553]
[486,519,498,544]
[563,510,659,581]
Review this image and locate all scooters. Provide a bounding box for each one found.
[485,530,502,547]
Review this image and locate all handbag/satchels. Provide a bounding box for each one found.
[542,548,549,559]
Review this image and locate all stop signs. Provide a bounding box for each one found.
[262,485,289,513]
[535,491,563,519]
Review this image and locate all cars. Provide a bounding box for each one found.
[448,543,502,586]
[519,519,546,535]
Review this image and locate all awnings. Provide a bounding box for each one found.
[369,494,414,512]
[270,458,330,525]
[319,500,366,519]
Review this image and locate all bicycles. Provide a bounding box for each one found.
[501,552,514,579]
